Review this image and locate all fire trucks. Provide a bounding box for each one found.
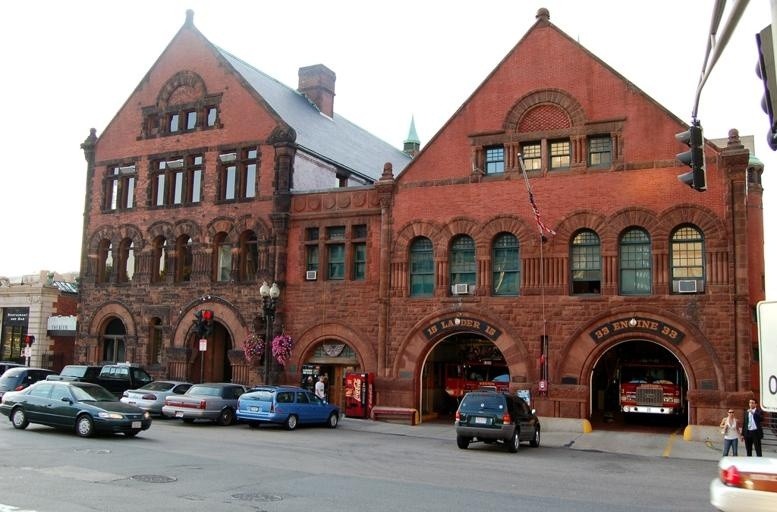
[443,357,510,397]
[611,354,685,422]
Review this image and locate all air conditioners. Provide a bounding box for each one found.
[306,271,316,279]
[679,280,697,293]
[455,284,469,294]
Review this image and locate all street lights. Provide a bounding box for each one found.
[260,282,280,383]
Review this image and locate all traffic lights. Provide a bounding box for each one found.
[755,59,776,151]
[204,311,213,339]
[674,129,693,190]
[193,312,201,336]
[20,337,26,348]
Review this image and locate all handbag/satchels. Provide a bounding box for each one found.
[721,426,728,435]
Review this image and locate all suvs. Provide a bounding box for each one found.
[455,392,541,452]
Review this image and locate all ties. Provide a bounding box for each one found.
[748,414,753,429]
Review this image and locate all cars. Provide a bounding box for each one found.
[708,455,777,511]
[0,380,151,438]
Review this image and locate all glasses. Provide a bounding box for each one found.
[728,412,734,413]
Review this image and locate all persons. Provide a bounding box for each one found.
[720,409,740,456]
[303,375,328,400]
[740,398,764,456]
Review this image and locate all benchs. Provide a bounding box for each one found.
[370,406,419,427]
